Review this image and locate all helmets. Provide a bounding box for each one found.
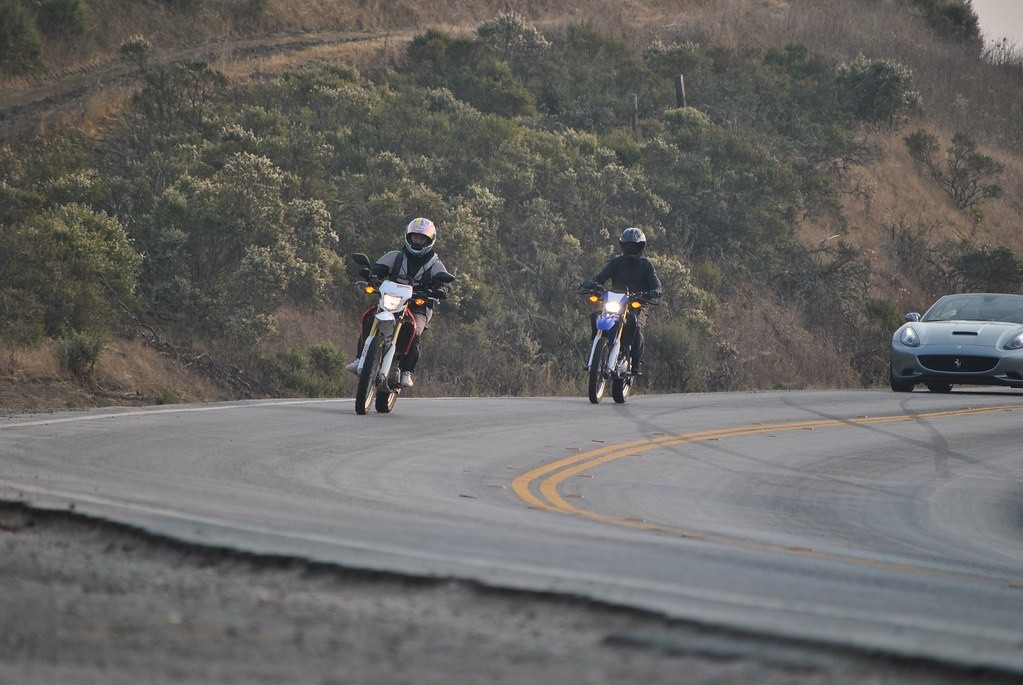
[619,228,647,261]
[405,218,437,258]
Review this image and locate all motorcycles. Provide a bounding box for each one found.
[578,280,661,406]
[348,252,456,415]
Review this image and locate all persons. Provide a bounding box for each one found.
[583,228,662,374]
[345,217,451,388]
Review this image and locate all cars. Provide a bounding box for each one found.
[890,292,1023,395]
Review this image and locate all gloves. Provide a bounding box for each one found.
[583,280,594,289]
[432,289,445,300]
[649,290,660,298]
[360,266,375,281]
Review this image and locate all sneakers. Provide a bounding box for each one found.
[401,371,414,387]
[345,359,360,375]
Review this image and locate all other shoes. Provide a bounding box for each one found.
[631,371,642,375]
[583,366,590,371]
